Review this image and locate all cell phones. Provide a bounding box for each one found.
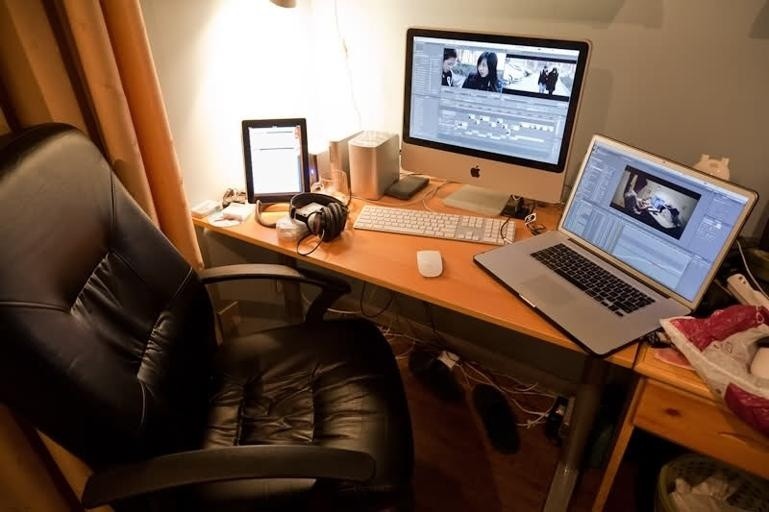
[645,331,671,348]
[388,174,429,200]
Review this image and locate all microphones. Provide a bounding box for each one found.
[346,190,352,205]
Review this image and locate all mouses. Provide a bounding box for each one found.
[417,250,442,278]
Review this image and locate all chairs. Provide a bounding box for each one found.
[0,122,421,511]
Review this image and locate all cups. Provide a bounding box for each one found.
[320,170,349,207]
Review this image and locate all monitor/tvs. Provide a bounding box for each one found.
[401,27,593,216]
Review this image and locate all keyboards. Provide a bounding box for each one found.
[353,205,514,246]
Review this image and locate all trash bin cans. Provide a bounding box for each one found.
[658,454,769,512]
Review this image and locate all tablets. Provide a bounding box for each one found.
[241,118,310,203]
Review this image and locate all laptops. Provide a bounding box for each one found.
[473,132,759,358]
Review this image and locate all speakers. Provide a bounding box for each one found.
[348,132,399,201]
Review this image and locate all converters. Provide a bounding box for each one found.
[440,350,459,368]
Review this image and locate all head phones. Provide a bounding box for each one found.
[289,192,348,241]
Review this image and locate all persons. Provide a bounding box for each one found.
[547,68,560,95]
[463,50,499,93]
[623,174,653,218]
[538,66,546,93]
[441,48,459,89]
